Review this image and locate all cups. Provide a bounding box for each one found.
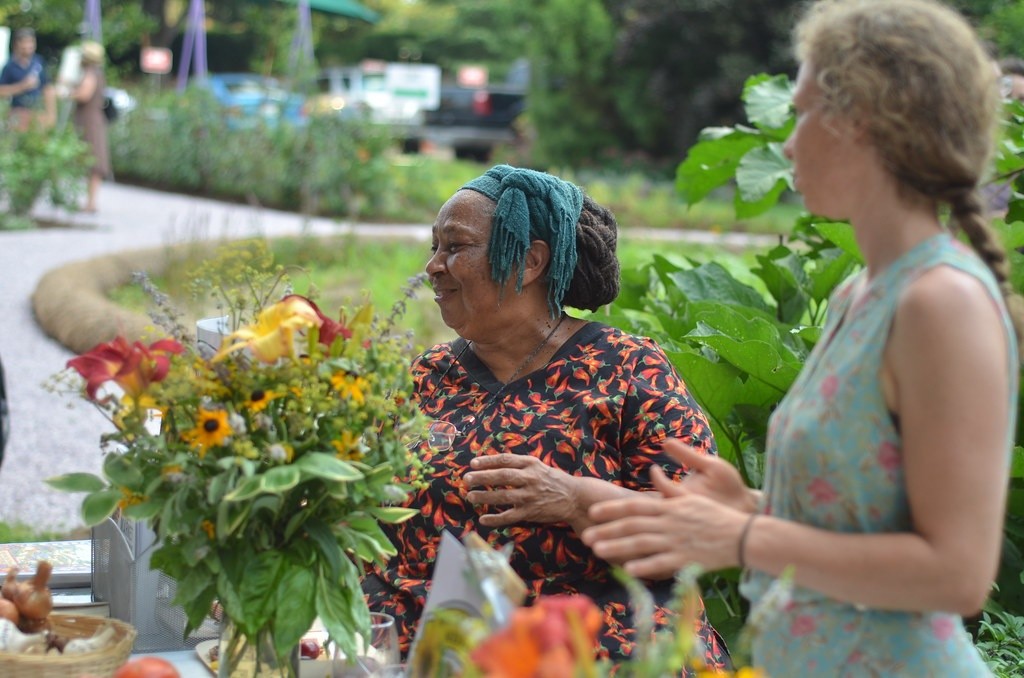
[332,612,401,678]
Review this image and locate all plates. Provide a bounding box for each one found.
[195,638,228,678]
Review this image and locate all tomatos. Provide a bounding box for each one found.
[114,656,179,678]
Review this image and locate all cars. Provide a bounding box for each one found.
[302,67,426,126]
[210,72,313,134]
[429,88,528,127]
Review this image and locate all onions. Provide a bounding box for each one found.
[0,561,52,629]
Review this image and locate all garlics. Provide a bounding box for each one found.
[0,616,116,656]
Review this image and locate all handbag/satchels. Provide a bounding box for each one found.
[102,97,118,123]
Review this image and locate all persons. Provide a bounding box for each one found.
[354,165,733,678]
[0,27,111,212]
[580,0,1018,677]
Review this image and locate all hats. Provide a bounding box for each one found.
[78,41,105,65]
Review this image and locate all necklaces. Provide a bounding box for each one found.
[392,312,565,512]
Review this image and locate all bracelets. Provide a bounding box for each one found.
[738,512,758,569]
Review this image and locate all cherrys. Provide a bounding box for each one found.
[300,641,320,660]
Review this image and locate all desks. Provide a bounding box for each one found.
[0,540,386,678]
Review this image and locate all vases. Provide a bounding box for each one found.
[217,617,300,678]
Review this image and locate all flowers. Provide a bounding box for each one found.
[51,243,456,662]
